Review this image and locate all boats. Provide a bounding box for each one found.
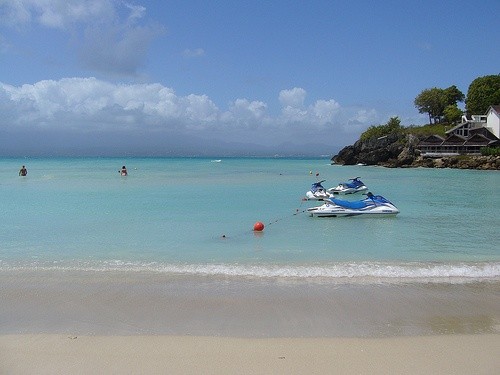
[309,192,401,218]
[328,176,367,195]
[305,180,335,200]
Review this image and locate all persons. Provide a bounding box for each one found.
[18,166,27,176]
[118,166,127,176]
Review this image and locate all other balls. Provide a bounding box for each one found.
[253,222,264,232]
